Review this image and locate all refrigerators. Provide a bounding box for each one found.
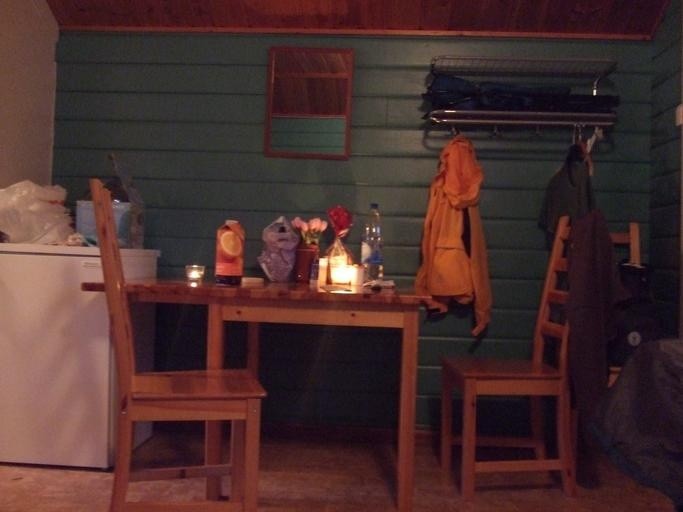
[1,240,163,471]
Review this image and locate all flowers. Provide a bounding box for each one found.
[291,218,329,242]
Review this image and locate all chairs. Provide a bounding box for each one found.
[441,217,598,497]
[605,216,642,390]
[85,172,269,512]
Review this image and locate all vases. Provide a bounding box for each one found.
[290,245,323,285]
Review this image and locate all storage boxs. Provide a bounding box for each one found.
[76,170,145,250]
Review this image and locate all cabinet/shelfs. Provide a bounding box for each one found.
[422,56,622,178]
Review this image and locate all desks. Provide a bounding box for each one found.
[80,277,435,512]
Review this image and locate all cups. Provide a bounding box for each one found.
[352,263,367,286]
[308,255,328,290]
[184,263,207,282]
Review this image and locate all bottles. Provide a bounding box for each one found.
[361,202,384,286]
